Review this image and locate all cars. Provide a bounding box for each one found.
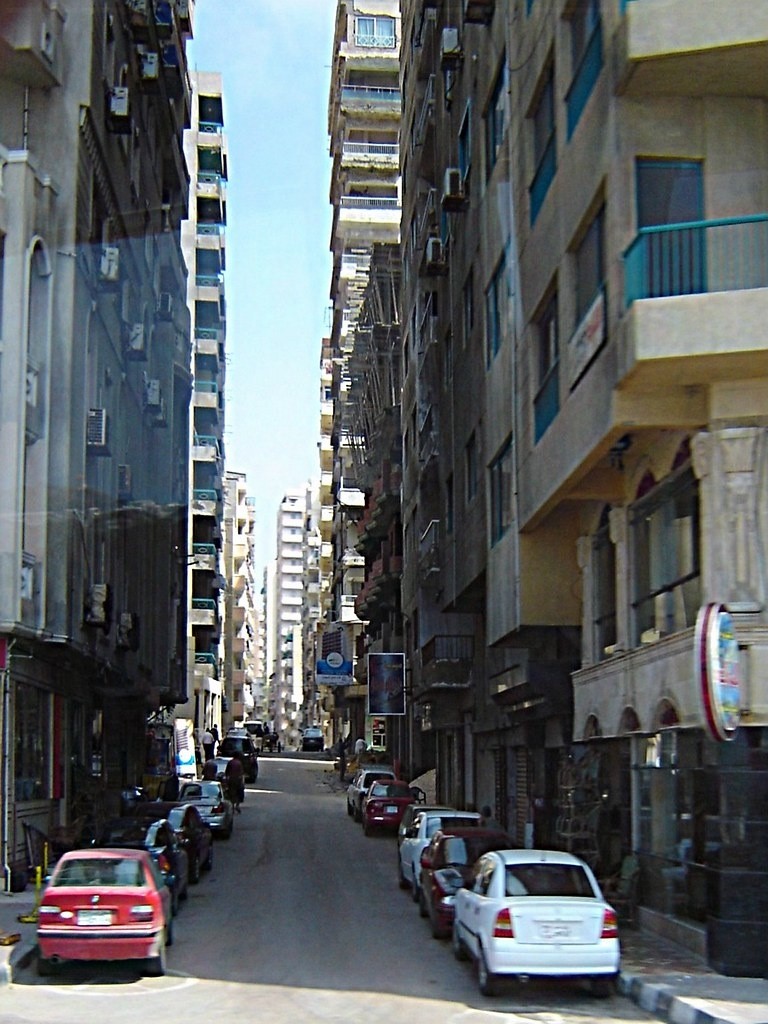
[75,816,190,917]
[397,803,454,848]
[359,779,416,835]
[209,756,233,791]
[133,801,214,871]
[37,847,176,975]
[302,728,325,749]
[398,811,483,902]
[450,850,621,996]
[180,779,233,837]
[218,728,258,784]
[419,827,521,940]
[346,767,395,821]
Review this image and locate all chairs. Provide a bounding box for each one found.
[598,853,642,930]
[25,816,85,865]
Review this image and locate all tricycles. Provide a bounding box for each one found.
[261,738,282,753]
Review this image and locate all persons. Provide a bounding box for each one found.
[180,807,210,881]
[264,723,279,747]
[354,737,367,755]
[223,758,246,815]
[479,804,506,831]
[194,724,223,764]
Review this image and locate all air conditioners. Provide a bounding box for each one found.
[118,610,140,649]
[126,323,147,363]
[83,581,112,630]
[111,85,132,135]
[119,463,136,506]
[439,164,462,212]
[101,248,122,293]
[427,237,444,272]
[155,293,176,323]
[441,28,460,74]
[144,55,159,78]
[85,407,110,457]
[145,380,171,427]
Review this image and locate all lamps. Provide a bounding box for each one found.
[641,629,660,645]
[604,642,628,654]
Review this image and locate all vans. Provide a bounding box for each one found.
[244,721,265,747]
